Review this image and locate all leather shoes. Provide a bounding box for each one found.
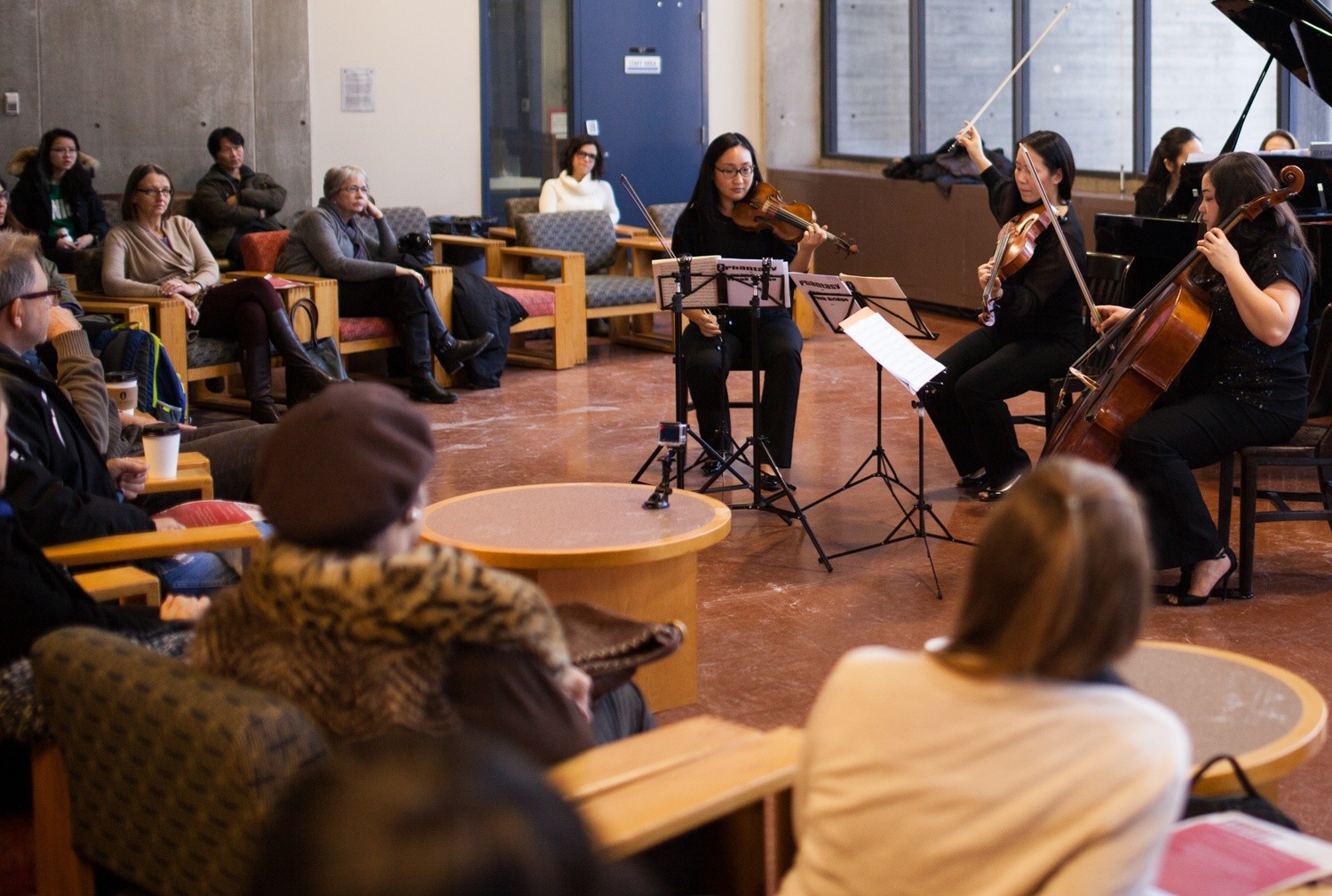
[760,473,780,488]
[704,452,727,476]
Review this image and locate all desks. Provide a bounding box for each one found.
[1116,640,1329,806]
[418,483,732,714]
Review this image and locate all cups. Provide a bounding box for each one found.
[142,422,181,480]
[105,371,137,415]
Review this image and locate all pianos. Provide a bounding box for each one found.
[1092,0,1332,424]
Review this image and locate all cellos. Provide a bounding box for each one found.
[1037,165,1304,470]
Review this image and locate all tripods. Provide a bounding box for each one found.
[629,260,979,603]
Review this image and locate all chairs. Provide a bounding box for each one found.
[0,191,1332,896]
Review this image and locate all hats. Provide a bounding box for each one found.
[249,383,436,544]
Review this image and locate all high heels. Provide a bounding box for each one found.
[1161,543,1239,606]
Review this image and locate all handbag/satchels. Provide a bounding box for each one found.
[1176,754,1300,832]
[287,297,348,402]
[557,601,684,704]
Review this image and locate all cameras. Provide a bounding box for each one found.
[657,424,688,446]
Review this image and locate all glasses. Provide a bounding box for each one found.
[0,287,63,309]
[134,187,174,199]
[576,150,596,163]
[339,184,370,195]
[715,164,756,179]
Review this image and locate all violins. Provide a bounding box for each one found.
[978,203,1059,327]
[733,181,860,260]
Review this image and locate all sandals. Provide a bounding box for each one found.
[977,468,1032,502]
[955,471,989,487]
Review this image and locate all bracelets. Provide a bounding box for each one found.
[193,282,202,289]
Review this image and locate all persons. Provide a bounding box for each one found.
[8,128,108,273]
[1133,126,1206,213]
[539,135,621,226]
[192,126,287,265]
[0,179,241,668]
[779,455,1193,896]
[186,381,662,768]
[1259,130,1302,151]
[1090,151,1319,606]
[670,132,829,491]
[915,120,1089,501]
[274,165,495,404]
[101,162,353,425]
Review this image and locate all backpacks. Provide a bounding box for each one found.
[96,320,186,425]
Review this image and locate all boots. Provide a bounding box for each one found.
[398,312,459,404]
[268,309,354,403]
[237,343,283,424]
[421,288,495,375]
